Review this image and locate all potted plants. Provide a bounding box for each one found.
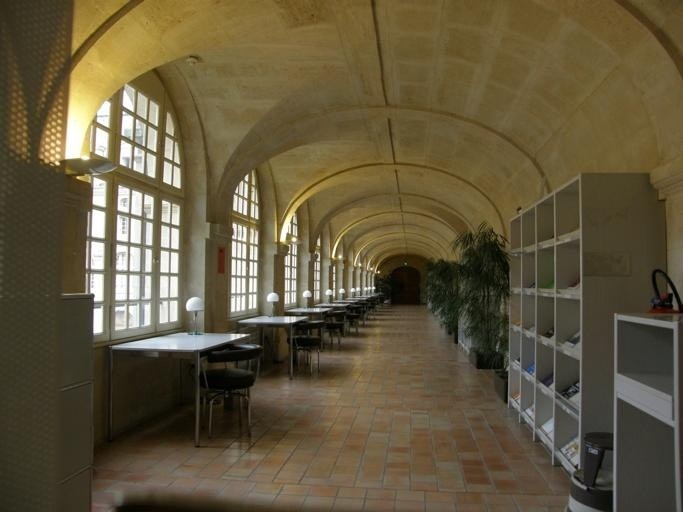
[418,220,514,370]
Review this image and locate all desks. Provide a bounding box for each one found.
[235,316,311,380]
[284,294,379,352]
[107,332,252,447]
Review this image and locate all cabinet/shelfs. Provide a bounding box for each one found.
[611,309,682,512]
[506,171,670,477]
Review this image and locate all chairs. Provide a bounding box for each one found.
[188,341,265,438]
[286,319,326,376]
[321,293,387,347]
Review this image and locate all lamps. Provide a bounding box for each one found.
[266,292,280,318]
[185,296,206,336]
[302,287,356,309]
